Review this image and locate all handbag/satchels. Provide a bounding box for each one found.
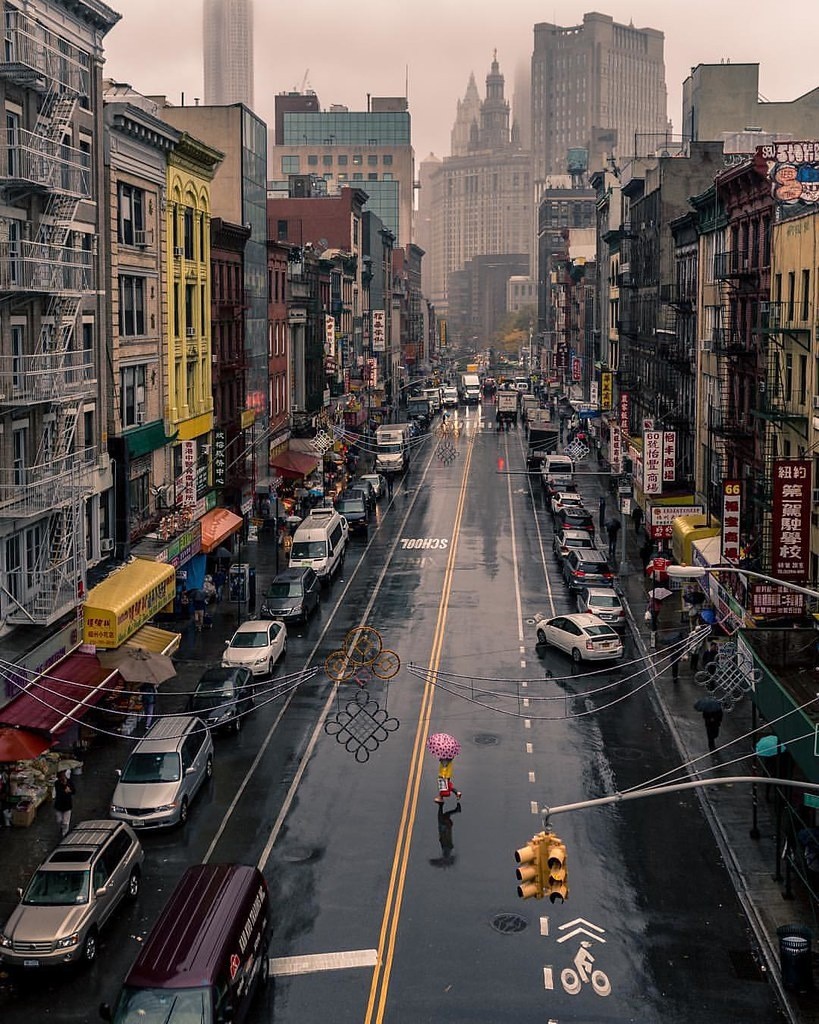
[651,606,656,611]
[645,610,652,620]
[439,778,451,797]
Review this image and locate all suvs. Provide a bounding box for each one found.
[259,567,321,636]
[0,818,146,969]
[545,479,577,505]
[110,717,215,833]
[562,550,614,594]
[554,507,595,538]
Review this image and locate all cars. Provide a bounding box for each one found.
[359,474,386,499]
[220,620,288,677]
[550,492,585,513]
[576,587,625,627]
[554,529,594,562]
[183,667,256,732]
[536,613,624,664]
[345,480,376,513]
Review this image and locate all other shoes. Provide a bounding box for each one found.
[457,792,461,800]
[434,798,443,802]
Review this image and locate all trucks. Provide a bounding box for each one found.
[364,350,566,475]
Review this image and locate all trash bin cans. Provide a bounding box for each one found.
[778,924,812,992]
[228,563,251,603]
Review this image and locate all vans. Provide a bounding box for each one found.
[337,489,368,531]
[287,507,349,584]
[540,455,574,486]
[97,862,273,1024]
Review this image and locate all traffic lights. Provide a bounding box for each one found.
[515,832,570,904]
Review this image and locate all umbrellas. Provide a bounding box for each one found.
[426,733,462,759]
[648,588,672,599]
[285,516,302,521]
[701,610,717,624]
[184,588,209,601]
[604,518,622,530]
[756,735,786,756]
[98,646,177,711]
[0,727,59,761]
[682,591,706,604]
[44,760,81,778]
[798,826,819,849]
[430,856,456,870]
[694,697,721,712]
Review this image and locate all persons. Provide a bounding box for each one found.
[599,495,723,691]
[434,758,462,802]
[703,712,723,749]
[291,522,297,529]
[214,573,225,602]
[764,754,819,888]
[284,534,292,560]
[385,470,394,493]
[53,772,76,837]
[261,415,380,528]
[139,683,157,729]
[438,802,461,857]
[193,600,205,632]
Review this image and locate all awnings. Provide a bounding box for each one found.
[271,450,318,479]
[0,651,118,739]
[195,508,243,553]
